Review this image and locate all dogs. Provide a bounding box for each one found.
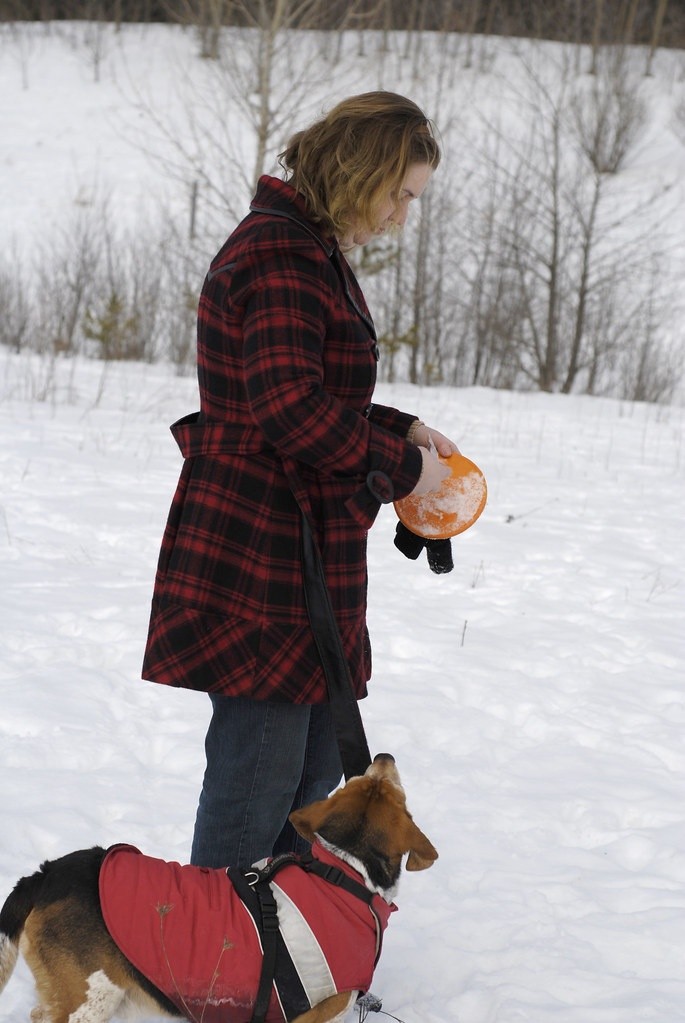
[1,753,438,1022]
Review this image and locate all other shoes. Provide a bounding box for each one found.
[357,991,382,1012]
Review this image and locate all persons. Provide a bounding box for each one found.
[142,91,460,1011]
